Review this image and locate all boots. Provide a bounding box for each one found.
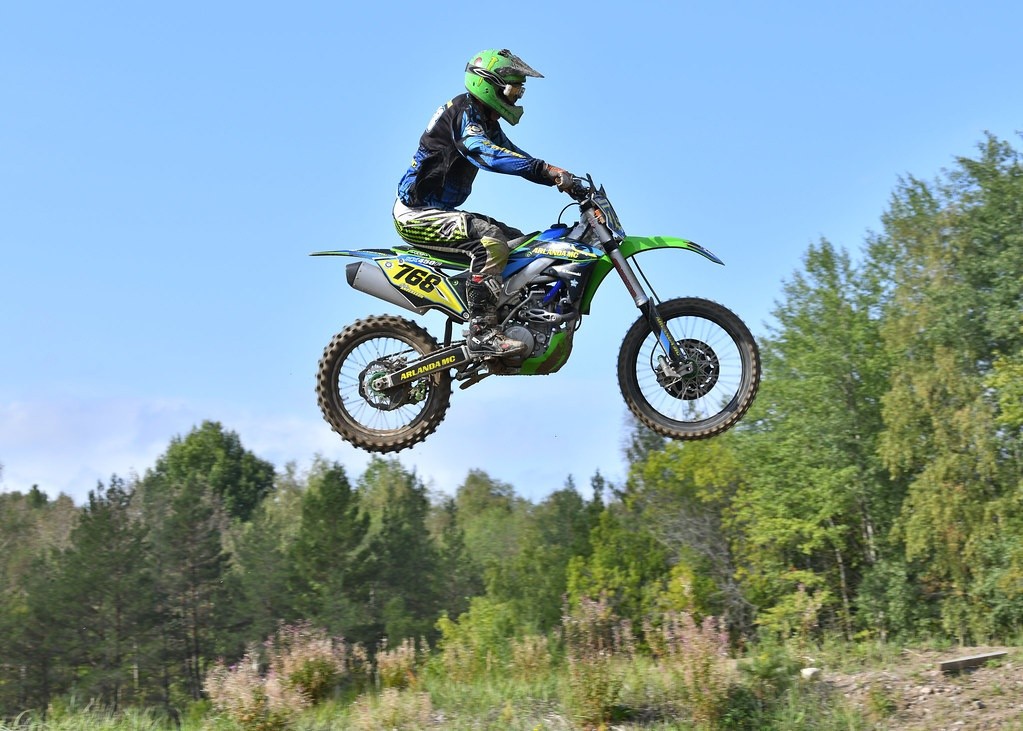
[467,272,528,355]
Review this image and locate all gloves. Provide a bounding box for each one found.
[541,163,573,192]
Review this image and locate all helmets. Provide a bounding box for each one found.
[465,48,545,126]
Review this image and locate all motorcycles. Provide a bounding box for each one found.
[309,171,763,454]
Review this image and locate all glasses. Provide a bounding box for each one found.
[500,82,525,103]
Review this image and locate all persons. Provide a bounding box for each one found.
[391,49,589,356]
